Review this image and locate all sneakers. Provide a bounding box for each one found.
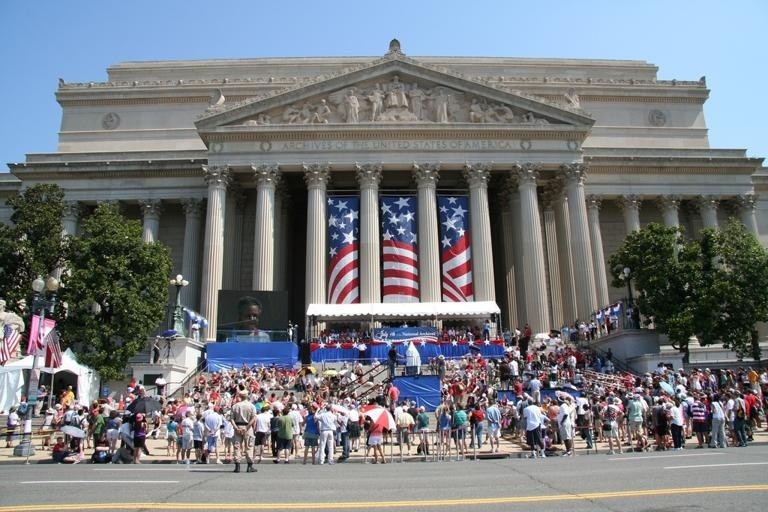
[166,450,387,465]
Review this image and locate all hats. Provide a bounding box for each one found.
[135,412,143,423]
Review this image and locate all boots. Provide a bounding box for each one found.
[234,463,240,473]
[247,463,257,472]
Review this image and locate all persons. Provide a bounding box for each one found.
[256,75,554,126]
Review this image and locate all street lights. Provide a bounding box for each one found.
[171,273,189,339]
[12,275,59,456]
[287,321,300,342]
[619,269,641,329]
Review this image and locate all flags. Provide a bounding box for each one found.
[0,325,23,366]
[438,195,474,302]
[326,195,360,304]
[380,196,420,302]
